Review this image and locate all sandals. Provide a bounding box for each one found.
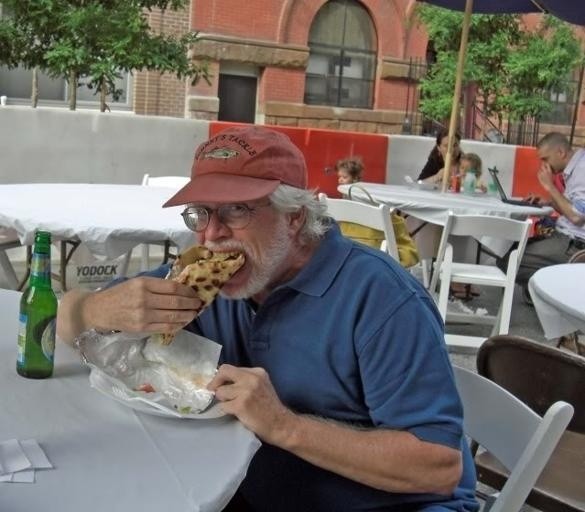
[450,286,474,301]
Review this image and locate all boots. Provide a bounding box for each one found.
[466,284,481,296]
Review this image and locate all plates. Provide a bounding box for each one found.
[459,187,483,195]
[89,362,230,421]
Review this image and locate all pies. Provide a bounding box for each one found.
[160,245,246,346]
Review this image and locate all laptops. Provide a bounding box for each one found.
[492,174,542,208]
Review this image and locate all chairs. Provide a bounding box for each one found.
[429,210,533,348]
[470,334,585,512]
[568,250,585,263]
[318,193,399,263]
[404,176,431,289]
[121,173,191,276]
[451,363,574,512]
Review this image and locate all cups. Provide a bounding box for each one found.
[465,170,480,194]
[487,181,498,194]
[450,176,461,194]
[432,177,441,190]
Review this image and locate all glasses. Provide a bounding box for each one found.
[181,199,275,231]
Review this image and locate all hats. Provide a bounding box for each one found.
[162,127,307,209]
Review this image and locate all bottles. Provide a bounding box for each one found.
[17,230,57,379]
[489,175,498,195]
[452,173,460,192]
[463,169,476,196]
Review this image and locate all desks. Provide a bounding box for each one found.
[0,184,199,291]
[0,287,262,512]
[336,182,554,302]
[528,263,585,355]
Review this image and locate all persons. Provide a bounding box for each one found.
[497,132,585,305]
[451,153,486,191]
[408,127,481,302]
[56,126,485,512]
[336,158,363,201]
